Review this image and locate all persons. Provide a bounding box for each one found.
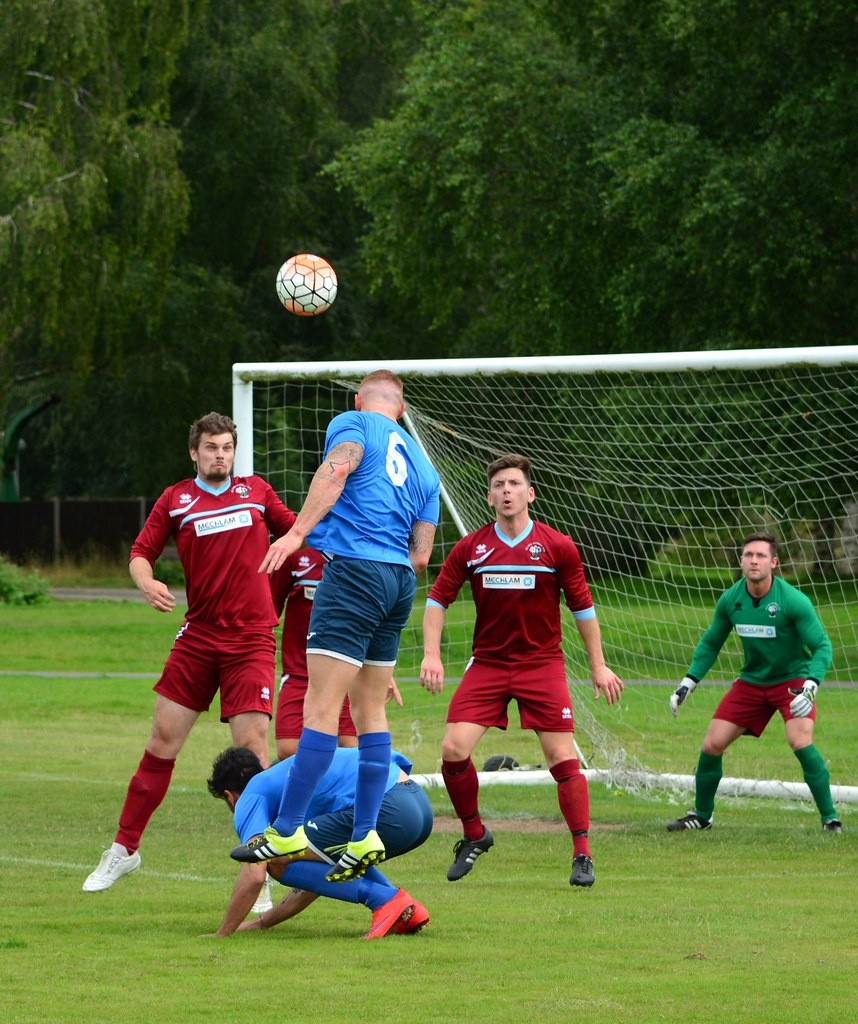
[664,532,842,833]
[268,545,403,760]
[81,410,297,914]
[420,453,623,887]
[206,745,434,940]
[229,369,442,882]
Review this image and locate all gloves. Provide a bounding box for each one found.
[787,679,819,719]
[670,677,697,717]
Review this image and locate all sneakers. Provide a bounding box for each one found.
[364,886,414,938]
[229,825,311,866]
[570,852,594,888]
[249,879,273,915]
[395,902,431,937]
[820,819,842,834]
[82,842,142,893]
[668,806,716,831]
[326,830,386,882]
[447,825,495,882]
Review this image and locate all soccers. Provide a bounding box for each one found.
[275,252,339,317]
